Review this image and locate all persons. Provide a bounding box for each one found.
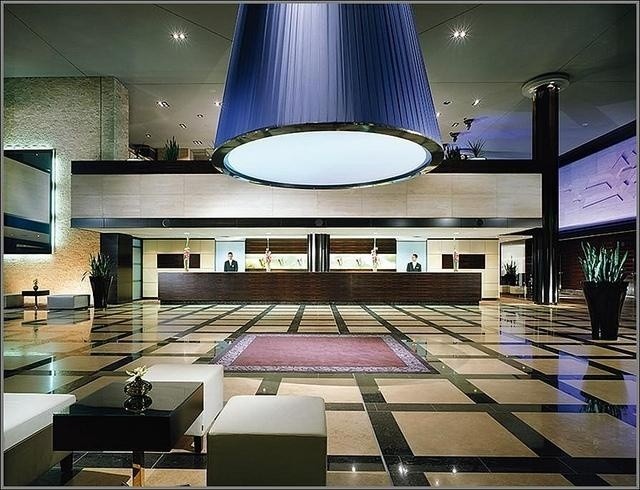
[406,254,421,272]
[224,252,238,272]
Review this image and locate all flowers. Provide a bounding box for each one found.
[452,249,460,262]
[183,240,191,269]
[370,246,381,267]
[264,247,272,263]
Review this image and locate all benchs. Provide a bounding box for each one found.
[46,293,91,311]
[3,294,25,309]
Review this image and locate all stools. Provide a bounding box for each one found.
[4,391,77,487]
[207,394,328,489]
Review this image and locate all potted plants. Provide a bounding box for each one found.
[466,137,488,160]
[81,252,120,308]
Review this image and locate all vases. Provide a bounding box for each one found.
[183,258,190,271]
[453,261,460,271]
[265,262,271,272]
[577,240,631,340]
[372,264,377,272]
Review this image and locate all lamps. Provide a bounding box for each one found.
[208,1,446,192]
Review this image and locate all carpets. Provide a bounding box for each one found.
[209,332,440,376]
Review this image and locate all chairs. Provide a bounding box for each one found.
[127,362,226,453]
